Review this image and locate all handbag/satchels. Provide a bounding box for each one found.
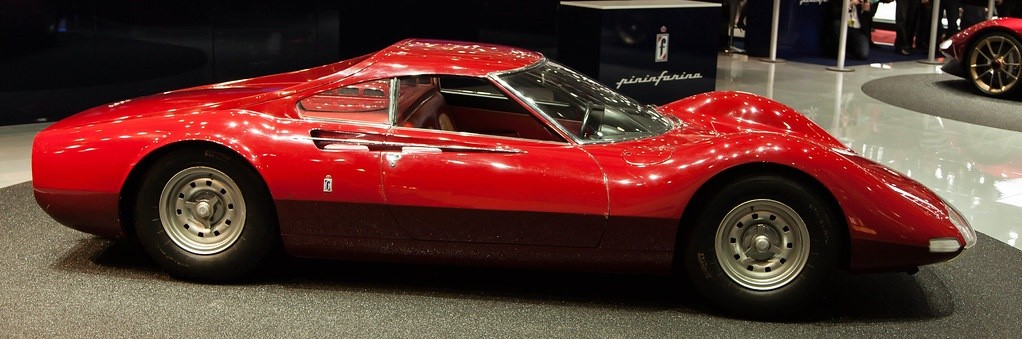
[847,4,861,29]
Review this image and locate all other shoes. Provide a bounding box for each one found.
[936,57,945,63]
[898,45,911,55]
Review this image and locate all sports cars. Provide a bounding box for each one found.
[938,17,1022,101]
[30,38,978,323]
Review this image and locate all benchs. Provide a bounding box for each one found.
[397,83,455,131]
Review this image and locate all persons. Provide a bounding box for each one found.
[744,0,1022,61]
